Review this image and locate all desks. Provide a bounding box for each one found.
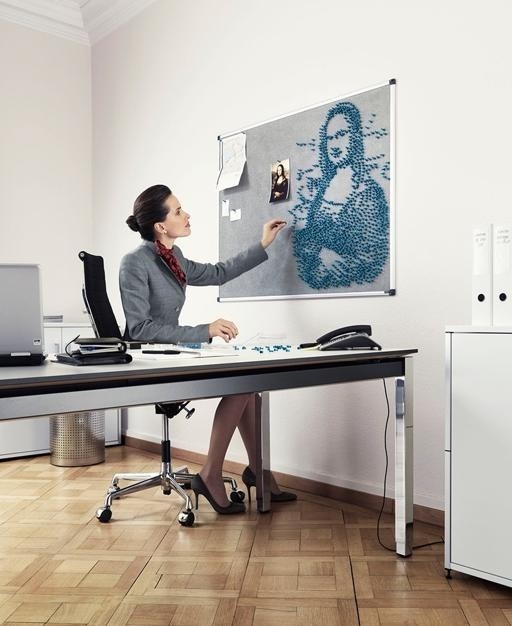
[0,347,421,557]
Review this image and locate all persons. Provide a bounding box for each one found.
[118,184,298,515]
[269,164,288,201]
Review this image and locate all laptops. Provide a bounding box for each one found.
[0,263,48,367]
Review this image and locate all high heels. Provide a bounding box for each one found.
[191,473,246,515]
[242,467,297,503]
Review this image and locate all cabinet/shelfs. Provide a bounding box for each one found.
[444,324,512,591]
[1,321,123,461]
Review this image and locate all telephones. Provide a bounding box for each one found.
[317,324,381,351]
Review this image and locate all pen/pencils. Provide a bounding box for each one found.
[142,350,181,354]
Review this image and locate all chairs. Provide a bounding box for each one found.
[78,251,246,526]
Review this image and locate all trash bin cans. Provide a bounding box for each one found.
[50,410,107,468]
